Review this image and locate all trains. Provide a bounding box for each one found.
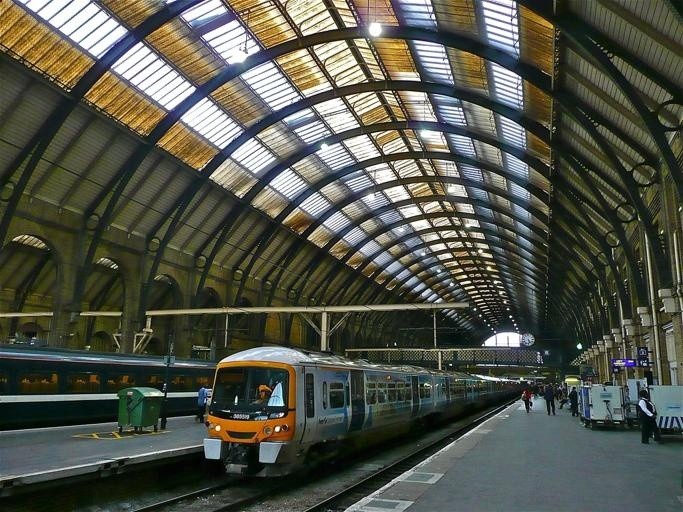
[204,347,520,478]
[0,344,218,431]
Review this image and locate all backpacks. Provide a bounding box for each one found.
[647,401,657,415]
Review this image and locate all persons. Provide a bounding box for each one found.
[569,387,578,417]
[521,381,569,415]
[249,385,272,406]
[196,385,208,423]
[638,390,662,443]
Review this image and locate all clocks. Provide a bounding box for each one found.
[522,333,536,348]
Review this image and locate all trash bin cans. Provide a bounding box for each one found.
[118,387,165,433]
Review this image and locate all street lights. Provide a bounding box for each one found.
[159,333,176,429]
